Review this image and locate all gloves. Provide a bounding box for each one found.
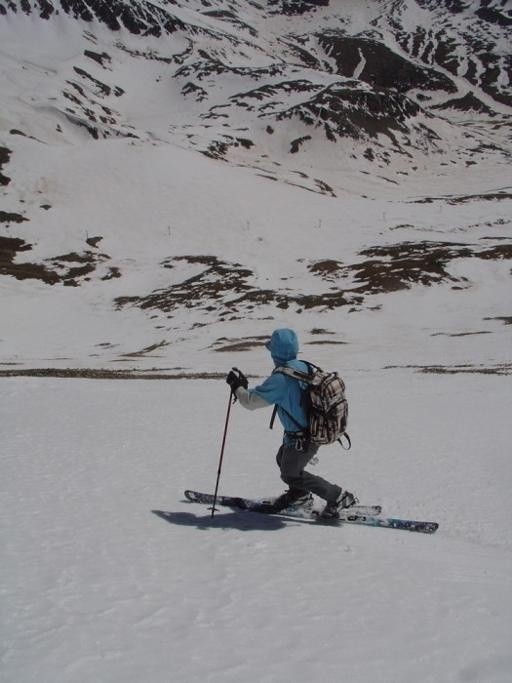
[270,359,352,451]
[227,368,250,395]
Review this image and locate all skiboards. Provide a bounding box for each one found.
[182,489,440,535]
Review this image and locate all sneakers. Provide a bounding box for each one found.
[275,486,311,508]
[320,491,357,521]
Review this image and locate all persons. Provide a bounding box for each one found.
[225,327,360,517]
